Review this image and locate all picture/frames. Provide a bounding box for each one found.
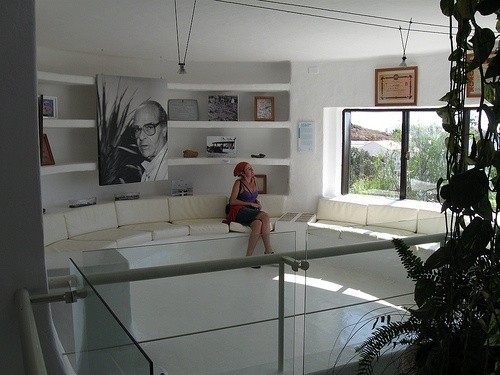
[42,96,58,120]
[255,175,267,194]
[375,66,418,106]
[254,96,275,121]
[465,51,498,98]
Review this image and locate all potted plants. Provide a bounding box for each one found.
[330,0,500,375]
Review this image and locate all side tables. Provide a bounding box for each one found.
[275,213,317,251]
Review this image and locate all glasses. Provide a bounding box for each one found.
[130,121,164,139]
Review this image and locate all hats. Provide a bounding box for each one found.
[234,162,247,177]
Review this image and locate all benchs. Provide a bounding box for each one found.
[307,198,455,253]
[43,193,285,249]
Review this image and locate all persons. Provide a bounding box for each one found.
[228,162,276,269]
[132,100,169,182]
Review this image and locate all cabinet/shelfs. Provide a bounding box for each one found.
[37,72,290,212]
